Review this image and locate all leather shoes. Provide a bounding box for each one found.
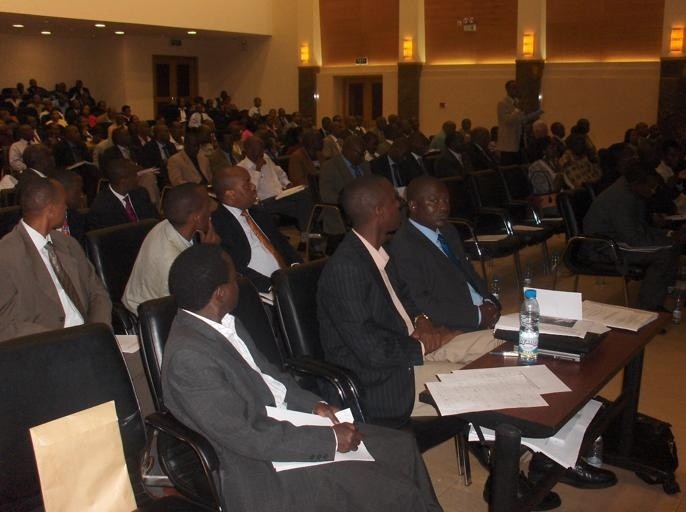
[529,459,618,489]
[483,477,560,511]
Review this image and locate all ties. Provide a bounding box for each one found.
[240,208,287,269]
[392,164,402,187]
[44,240,87,316]
[351,164,361,177]
[122,194,138,223]
[436,232,481,298]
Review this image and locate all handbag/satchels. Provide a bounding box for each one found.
[597,395,679,474]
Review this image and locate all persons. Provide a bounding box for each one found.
[160,242,445,511]
[392,175,617,489]
[315,175,562,511]
[1,80,686,339]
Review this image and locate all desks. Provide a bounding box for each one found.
[419,298,680,511]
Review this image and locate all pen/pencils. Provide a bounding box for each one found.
[488,351,519,357]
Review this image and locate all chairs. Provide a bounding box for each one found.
[0,322,223,512]
[268,260,472,485]
[137,284,355,422]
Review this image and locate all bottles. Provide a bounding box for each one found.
[519,290,539,364]
